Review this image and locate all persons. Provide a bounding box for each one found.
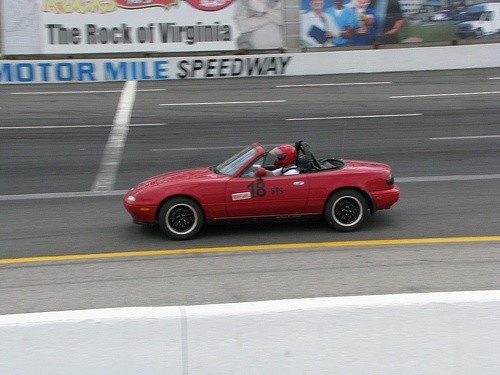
[299,0,405,48]
[250,144,301,177]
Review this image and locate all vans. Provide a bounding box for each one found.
[456,3,500,39]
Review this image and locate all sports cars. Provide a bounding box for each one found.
[123,144,400,232]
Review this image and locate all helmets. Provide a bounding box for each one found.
[274,145,296,167]
